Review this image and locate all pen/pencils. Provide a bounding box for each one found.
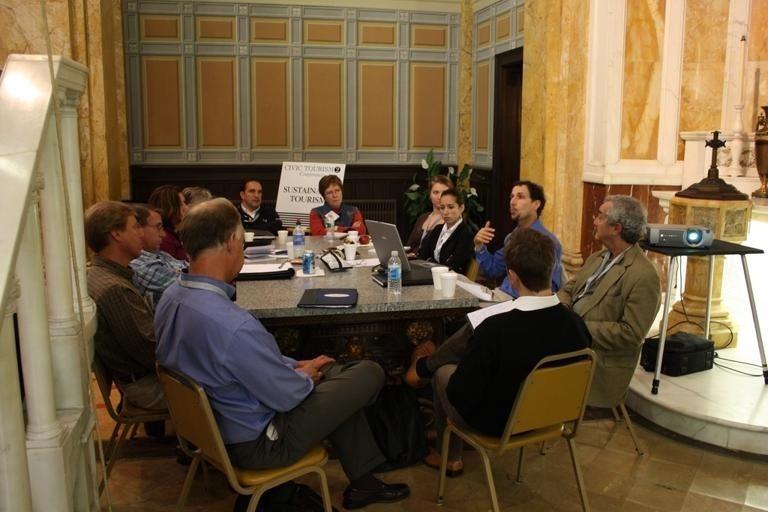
[279,261,289,270]
[414,249,419,256]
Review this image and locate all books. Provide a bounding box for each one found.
[372,271,432,288]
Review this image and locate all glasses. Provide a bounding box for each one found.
[147,223,163,230]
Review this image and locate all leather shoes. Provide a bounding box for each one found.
[342,478,411,510]
[422,449,464,477]
[403,340,437,389]
[175,447,193,465]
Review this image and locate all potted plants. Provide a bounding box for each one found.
[400,147,485,227]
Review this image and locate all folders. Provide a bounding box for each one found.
[243,230,276,242]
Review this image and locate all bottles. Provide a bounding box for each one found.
[293,221,305,258]
[387,251,401,294]
[325,214,335,241]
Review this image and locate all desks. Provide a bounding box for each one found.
[236,236,479,327]
[639,224,768,395]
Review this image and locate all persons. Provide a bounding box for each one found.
[310,176,367,237]
[147,184,189,264]
[234,181,283,236]
[155,198,409,512]
[404,176,454,255]
[474,180,566,306]
[181,186,210,207]
[83,198,214,472]
[127,201,189,306]
[404,228,593,478]
[417,191,476,273]
[558,194,661,410]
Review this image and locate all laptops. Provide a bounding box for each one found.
[364,221,452,273]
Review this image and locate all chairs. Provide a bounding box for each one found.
[436,349,598,512]
[152,373,332,512]
[91,359,183,499]
[541,390,644,456]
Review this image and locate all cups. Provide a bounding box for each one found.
[243,232,254,244]
[286,242,295,259]
[343,242,357,261]
[348,231,359,241]
[440,273,458,298]
[277,230,289,243]
[430,267,450,291]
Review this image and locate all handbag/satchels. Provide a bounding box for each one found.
[639,328,718,377]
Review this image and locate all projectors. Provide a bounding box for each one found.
[646,225,716,249]
